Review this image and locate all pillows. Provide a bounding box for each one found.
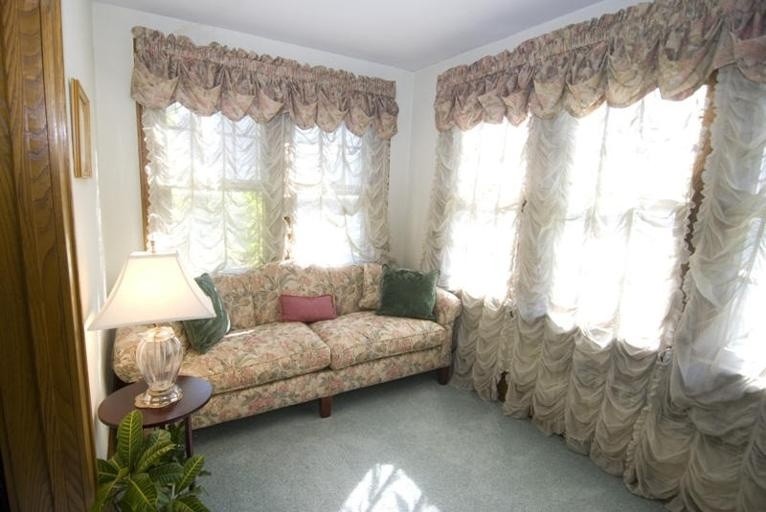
[185,271,231,353]
[375,263,442,321]
[279,294,337,321]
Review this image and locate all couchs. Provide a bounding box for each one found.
[112,261,463,435]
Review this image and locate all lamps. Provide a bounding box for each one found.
[86,237,217,409]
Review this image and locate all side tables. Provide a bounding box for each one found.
[96,374,213,494]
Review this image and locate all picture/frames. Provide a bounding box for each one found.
[67,74,93,182]
